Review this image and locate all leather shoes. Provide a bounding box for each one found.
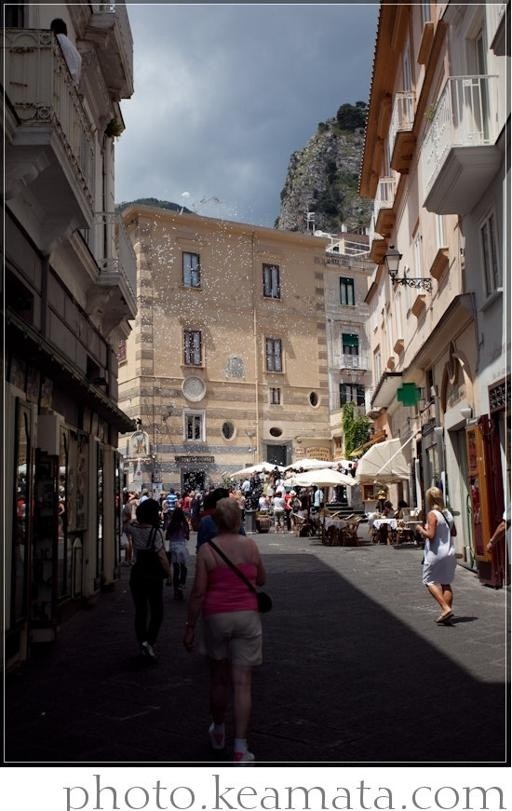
[142,641,156,657]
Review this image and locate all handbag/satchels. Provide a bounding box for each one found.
[256,590,272,612]
[135,549,170,578]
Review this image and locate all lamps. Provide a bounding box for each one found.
[431,384,440,399]
[459,407,474,419]
[384,244,432,294]
[434,426,444,437]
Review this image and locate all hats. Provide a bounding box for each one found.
[289,490,297,497]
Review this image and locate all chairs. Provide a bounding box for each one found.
[290,505,425,548]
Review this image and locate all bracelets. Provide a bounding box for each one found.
[490,538,495,545]
[185,619,197,629]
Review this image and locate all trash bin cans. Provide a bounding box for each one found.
[245,510,256,532]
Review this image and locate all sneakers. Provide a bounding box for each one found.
[232,751,254,760]
[209,722,224,749]
[178,584,186,591]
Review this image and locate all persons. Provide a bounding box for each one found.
[120,462,410,534]
[485,507,511,566]
[197,488,246,554]
[118,491,134,567]
[129,498,171,657]
[183,497,266,761]
[166,507,189,590]
[415,486,458,623]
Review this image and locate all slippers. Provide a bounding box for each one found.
[435,612,454,625]
